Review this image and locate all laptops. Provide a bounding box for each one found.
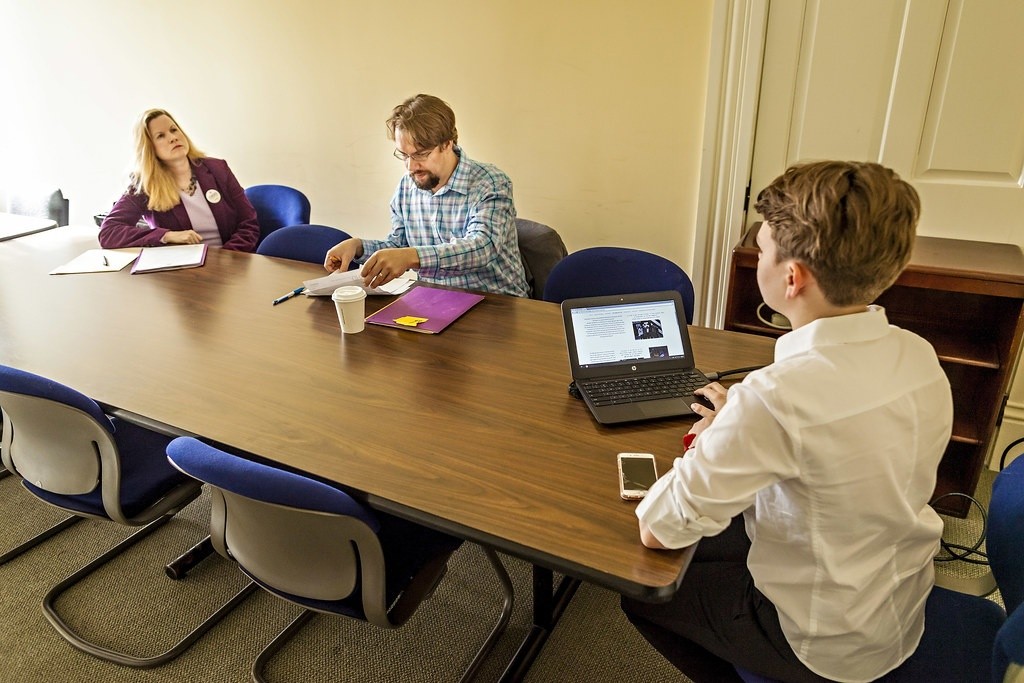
[561,290,716,426]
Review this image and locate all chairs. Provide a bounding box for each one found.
[0,186,695,683]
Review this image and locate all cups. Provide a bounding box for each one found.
[331,285,366,335]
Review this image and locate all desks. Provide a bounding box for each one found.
[1,217,54,241]
[0,226,781,683]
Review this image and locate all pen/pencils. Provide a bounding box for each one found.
[103,256,107,266]
[273,287,305,305]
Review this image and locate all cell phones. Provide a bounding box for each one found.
[617,452,658,501]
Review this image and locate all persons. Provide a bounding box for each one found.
[636,322,662,338]
[621,161,954,683]
[324,94,531,300]
[98,108,260,252]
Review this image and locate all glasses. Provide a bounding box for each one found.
[394,145,439,162]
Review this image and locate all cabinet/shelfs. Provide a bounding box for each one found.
[721,223,1024,520]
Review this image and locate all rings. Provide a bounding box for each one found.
[379,273,386,278]
